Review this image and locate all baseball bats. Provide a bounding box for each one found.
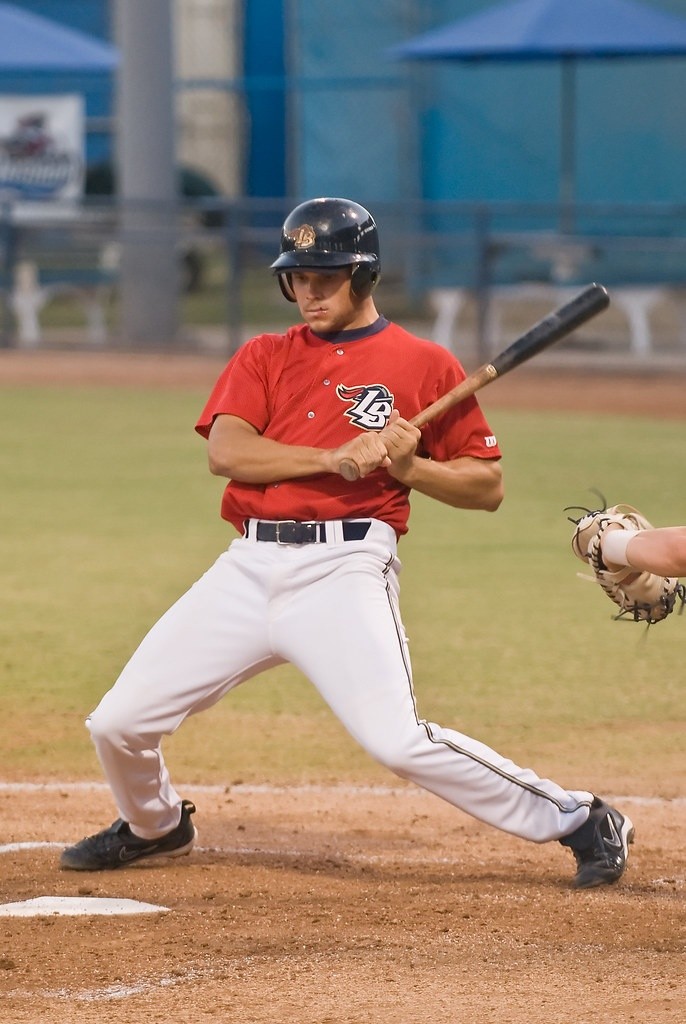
[338,284,609,482]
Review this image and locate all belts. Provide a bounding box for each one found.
[244,518,372,545]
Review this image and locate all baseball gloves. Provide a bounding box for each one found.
[570,501,679,625]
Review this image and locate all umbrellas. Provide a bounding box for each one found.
[393,0,686,239]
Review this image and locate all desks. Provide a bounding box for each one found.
[426,281,665,360]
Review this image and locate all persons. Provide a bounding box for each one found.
[59,198,635,890]
[564,504,686,625]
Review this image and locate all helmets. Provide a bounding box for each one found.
[270,197,381,304]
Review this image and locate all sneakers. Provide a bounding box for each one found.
[58,800,199,871]
[559,794,636,890]
